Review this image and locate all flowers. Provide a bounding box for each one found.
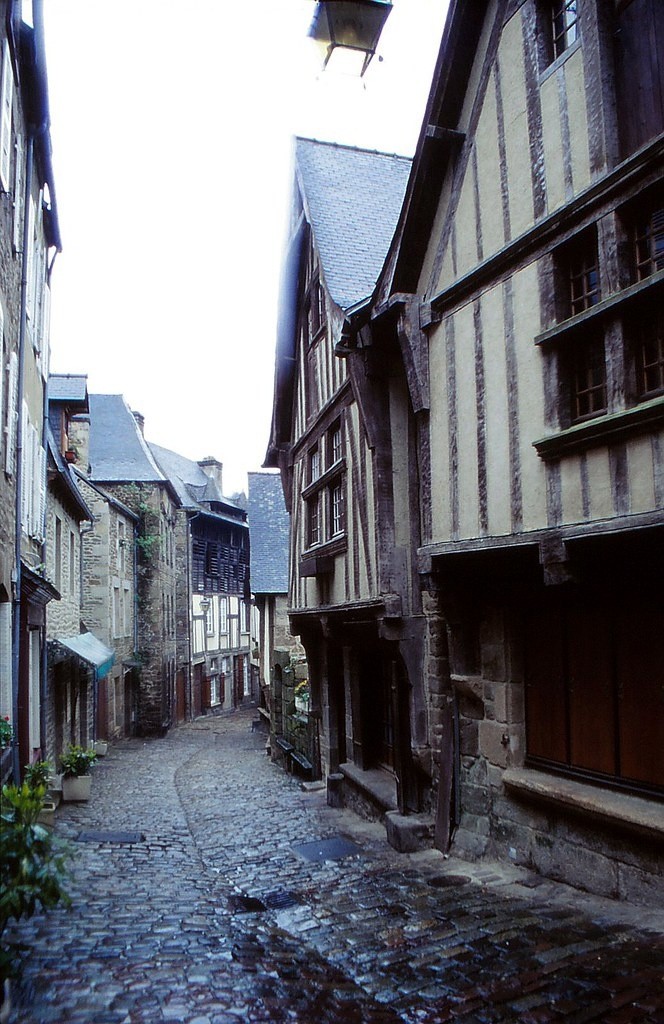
[0,716,14,751]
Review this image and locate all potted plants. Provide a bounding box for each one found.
[65,445,78,464]
[58,744,96,801]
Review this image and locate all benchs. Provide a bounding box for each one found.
[276,738,312,782]
